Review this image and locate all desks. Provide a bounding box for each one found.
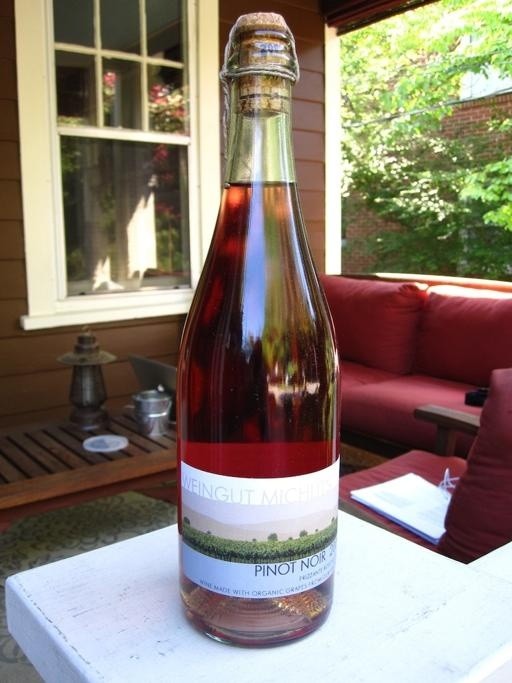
[0,403,180,563]
[2,508,511,683]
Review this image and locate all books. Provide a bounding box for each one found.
[351,471,456,544]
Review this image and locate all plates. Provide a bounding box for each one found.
[82,431,130,454]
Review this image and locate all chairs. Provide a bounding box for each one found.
[332,367,512,564]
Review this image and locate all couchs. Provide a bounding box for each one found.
[314,268,511,450]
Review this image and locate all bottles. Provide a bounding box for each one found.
[176,8,340,645]
